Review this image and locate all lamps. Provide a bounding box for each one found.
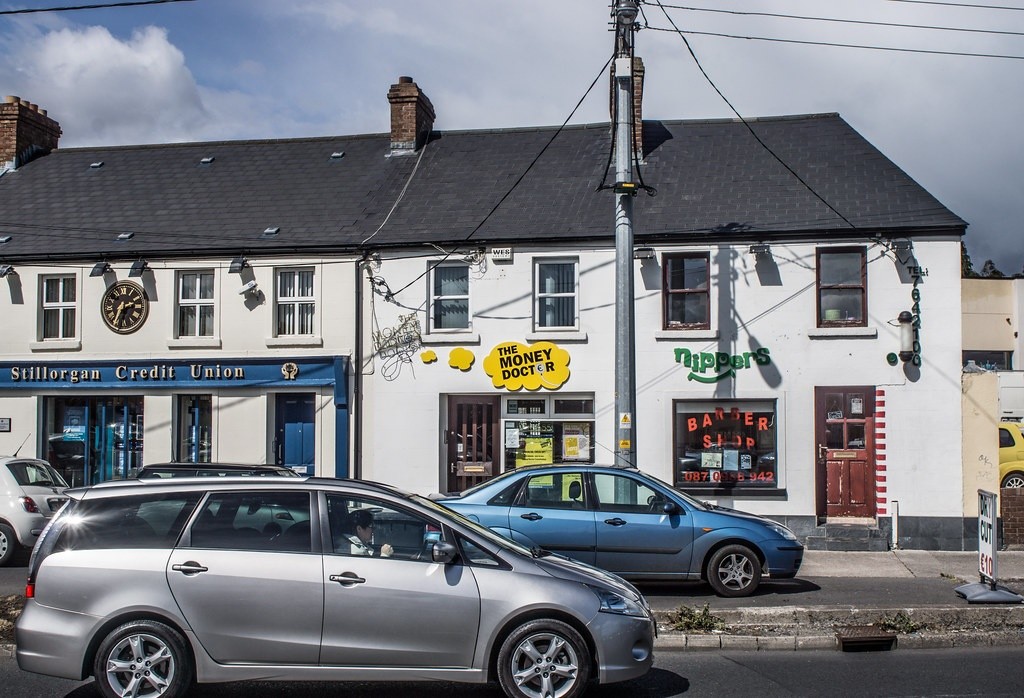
[898,310,914,362]
[128,260,150,277]
[228,259,250,274]
[749,246,769,253]
[891,241,908,249]
[89,263,114,278]
[0,265,17,278]
[634,248,655,260]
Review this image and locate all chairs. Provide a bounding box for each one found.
[568,480,586,511]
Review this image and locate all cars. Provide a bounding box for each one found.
[680,450,777,483]
[49,431,127,486]
[124,462,309,550]
[0,455,71,563]
[14,474,655,698]
[422,461,806,598]
[997,421,1024,487]
[452,432,494,491]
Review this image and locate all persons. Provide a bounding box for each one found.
[335,509,394,558]
